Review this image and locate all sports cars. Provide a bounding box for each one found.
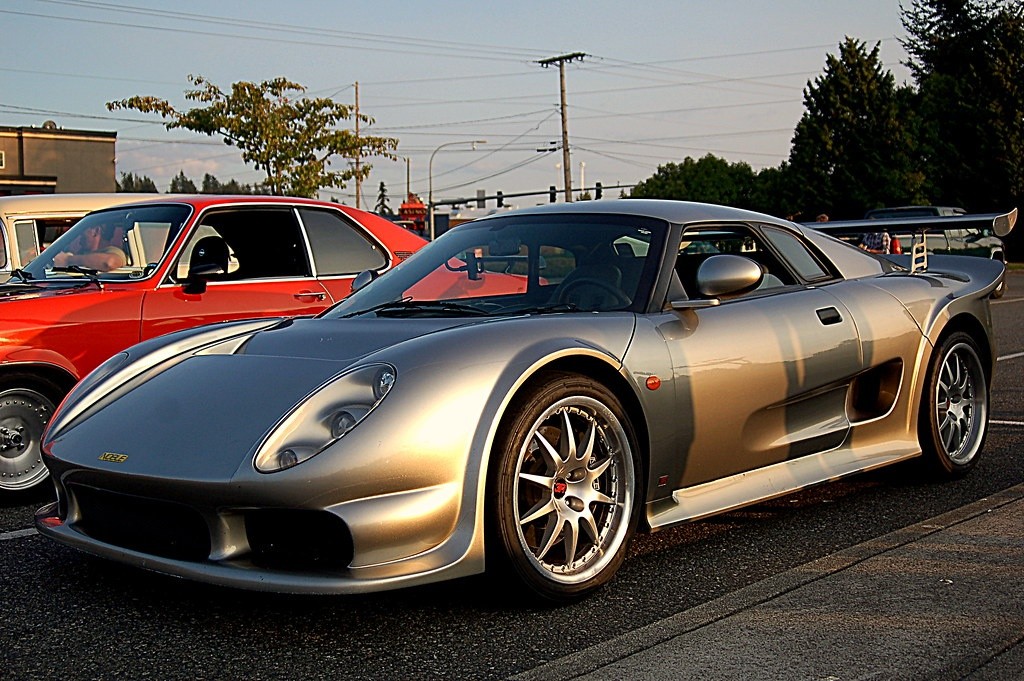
[33,199,1017,605]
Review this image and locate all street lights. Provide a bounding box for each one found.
[429,141,487,243]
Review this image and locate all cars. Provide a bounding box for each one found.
[0,195,548,510]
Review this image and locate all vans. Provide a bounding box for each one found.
[0,193,239,284]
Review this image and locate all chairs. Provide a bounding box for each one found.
[543,242,634,310]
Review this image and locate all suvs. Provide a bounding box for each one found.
[860,206,1008,299]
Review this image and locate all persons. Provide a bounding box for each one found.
[816,215,828,221]
[38,211,126,271]
[859,218,891,254]
